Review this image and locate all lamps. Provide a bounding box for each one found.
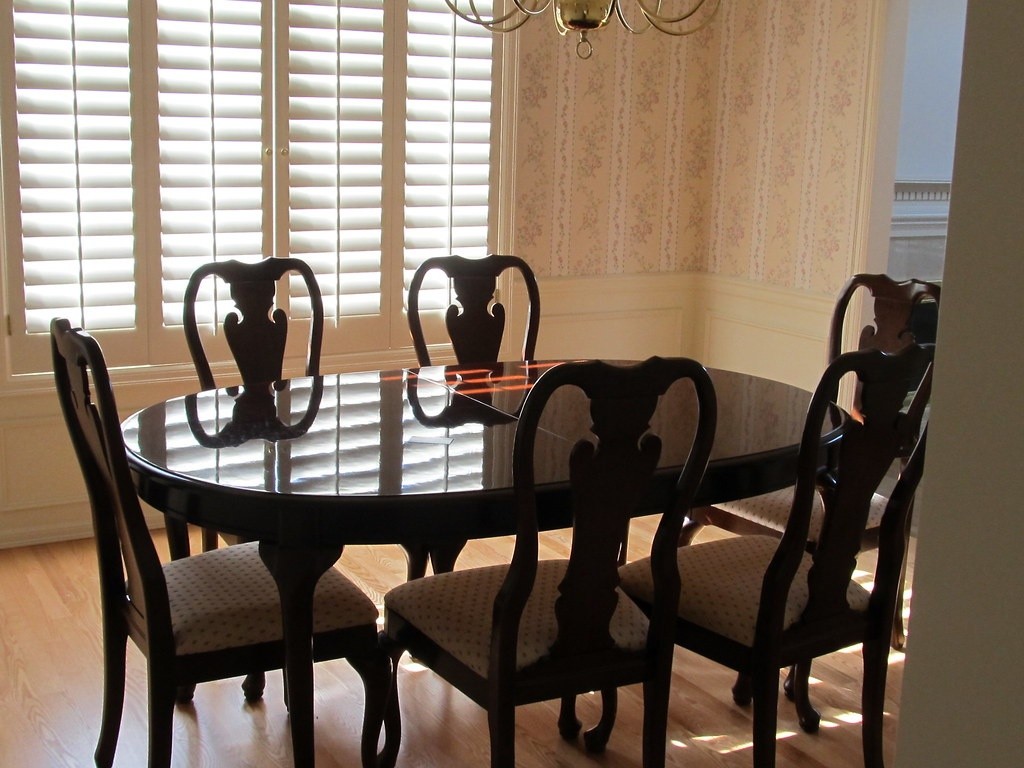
[444,0,721,60]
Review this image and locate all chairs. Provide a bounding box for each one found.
[377,356,720,768]
[407,254,541,577]
[677,272,941,701]
[183,256,326,703]
[615,342,937,768]
[49,317,387,768]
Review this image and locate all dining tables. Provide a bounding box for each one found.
[119,357,857,768]
